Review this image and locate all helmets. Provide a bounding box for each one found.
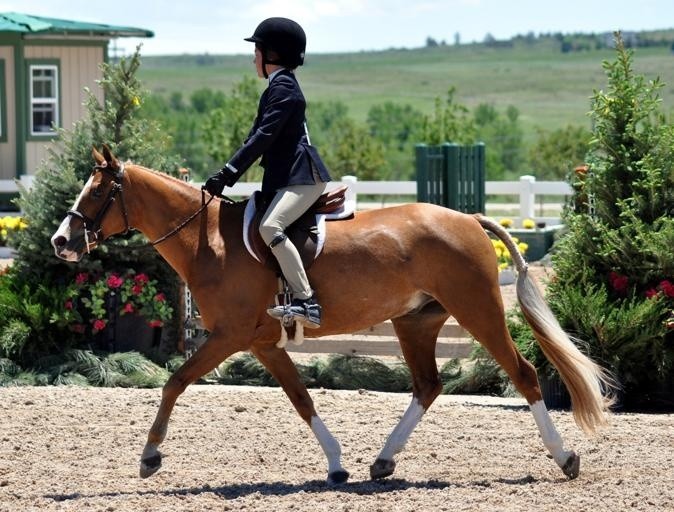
[243,17,306,65]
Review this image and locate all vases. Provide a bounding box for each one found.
[498,265,516,285]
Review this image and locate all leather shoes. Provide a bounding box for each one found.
[266,303,321,329]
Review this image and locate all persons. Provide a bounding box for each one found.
[205,17,332,330]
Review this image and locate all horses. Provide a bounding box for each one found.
[51,144,622,489]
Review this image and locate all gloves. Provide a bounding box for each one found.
[205,175,226,196]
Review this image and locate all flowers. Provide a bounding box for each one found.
[491,237,529,271]
